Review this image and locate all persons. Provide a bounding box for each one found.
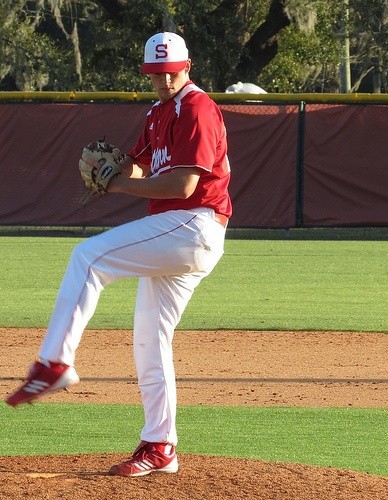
[5,32,233,476]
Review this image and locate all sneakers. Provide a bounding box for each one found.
[6,361,80,405]
[109,441,178,476]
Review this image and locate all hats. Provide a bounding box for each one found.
[141,32,189,74]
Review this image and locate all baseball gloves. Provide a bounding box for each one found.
[78,145,124,199]
